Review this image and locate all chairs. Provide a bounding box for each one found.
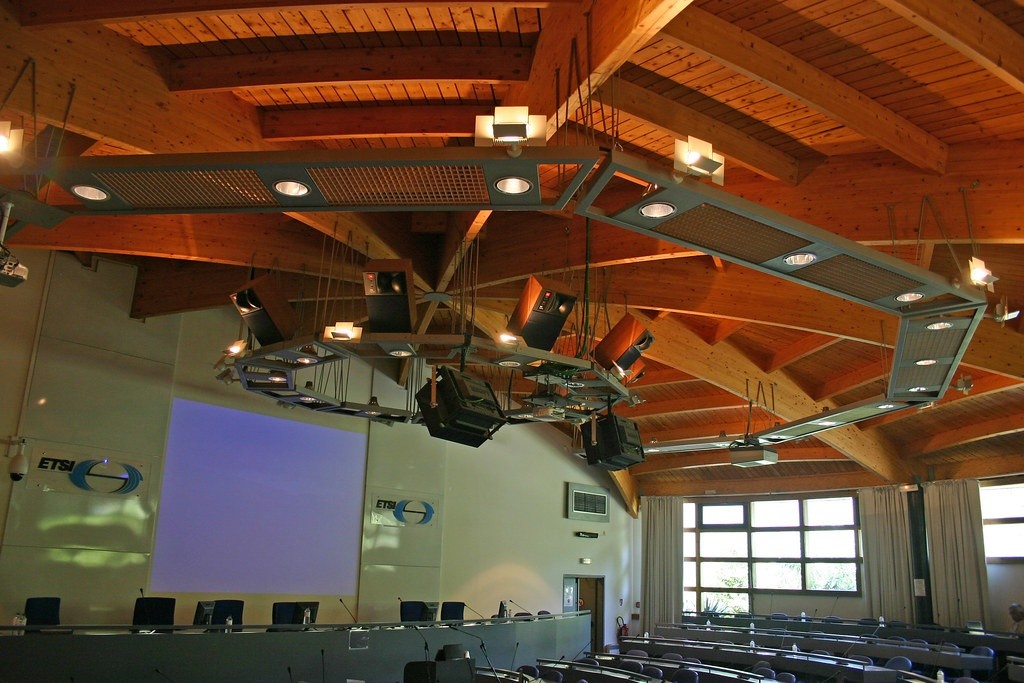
[24,597,1024,683]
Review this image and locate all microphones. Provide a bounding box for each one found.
[777,621,789,656]
[287,666,293,683]
[572,638,595,661]
[414,626,429,651]
[828,595,839,622]
[737,652,783,679]
[398,597,410,629]
[449,627,486,651]
[511,642,519,671]
[613,633,640,660]
[462,603,484,624]
[140,588,160,633]
[950,598,962,633]
[836,633,863,664]
[339,599,369,629]
[155,668,174,683]
[509,600,535,621]
[804,609,818,638]
[321,649,325,683]
[821,670,841,683]
[539,655,565,683]
[925,639,945,683]
[766,591,773,620]
[887,606,906,628]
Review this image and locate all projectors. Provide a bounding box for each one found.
[729,446,778,468]
[0,260,29,288]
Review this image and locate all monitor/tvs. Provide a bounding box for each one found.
[498,599,508,623]
[283,602,319,631]
[193,601,218,633]
[418,602,439,627]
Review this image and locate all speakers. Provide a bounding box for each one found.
[621,358,648,387]
[362,259,417,333]
[505,273,577,352]
[228,273,298,347]
[589,312,656,378]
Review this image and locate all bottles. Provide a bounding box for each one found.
[13,613,26,637]
[792,643,798,657]
[508,609,512,623]
[750,622,754,634]
[879,615,884,627]
[706,619,710,630]
[750,640,755,653]
[303,607,310,624]
[504,608,507,618]
[937,669,944,683]
[644,631,649,643]
[225,616,232,633]
[801,611,806,622]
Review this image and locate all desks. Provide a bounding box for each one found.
[0,610,1024,683]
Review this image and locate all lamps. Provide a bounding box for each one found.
[466,108,556,155]
[949,377,973,395]
[216,301,648,416]
[984,295,1020,330]
[667,132,729,186]
[0,117,33,170]
[965,254,997,295]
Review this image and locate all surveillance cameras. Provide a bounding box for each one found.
[8,444,28,482]
